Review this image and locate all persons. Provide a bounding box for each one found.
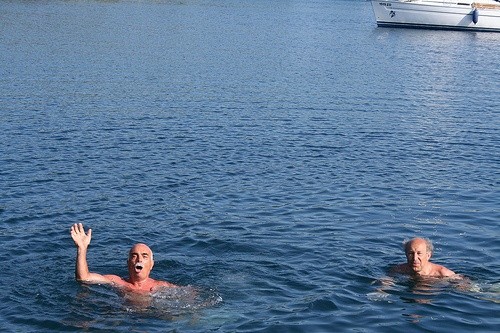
[376,237,500,325]
[70,222,211,319]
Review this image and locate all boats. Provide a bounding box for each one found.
[369,0,500,32]
